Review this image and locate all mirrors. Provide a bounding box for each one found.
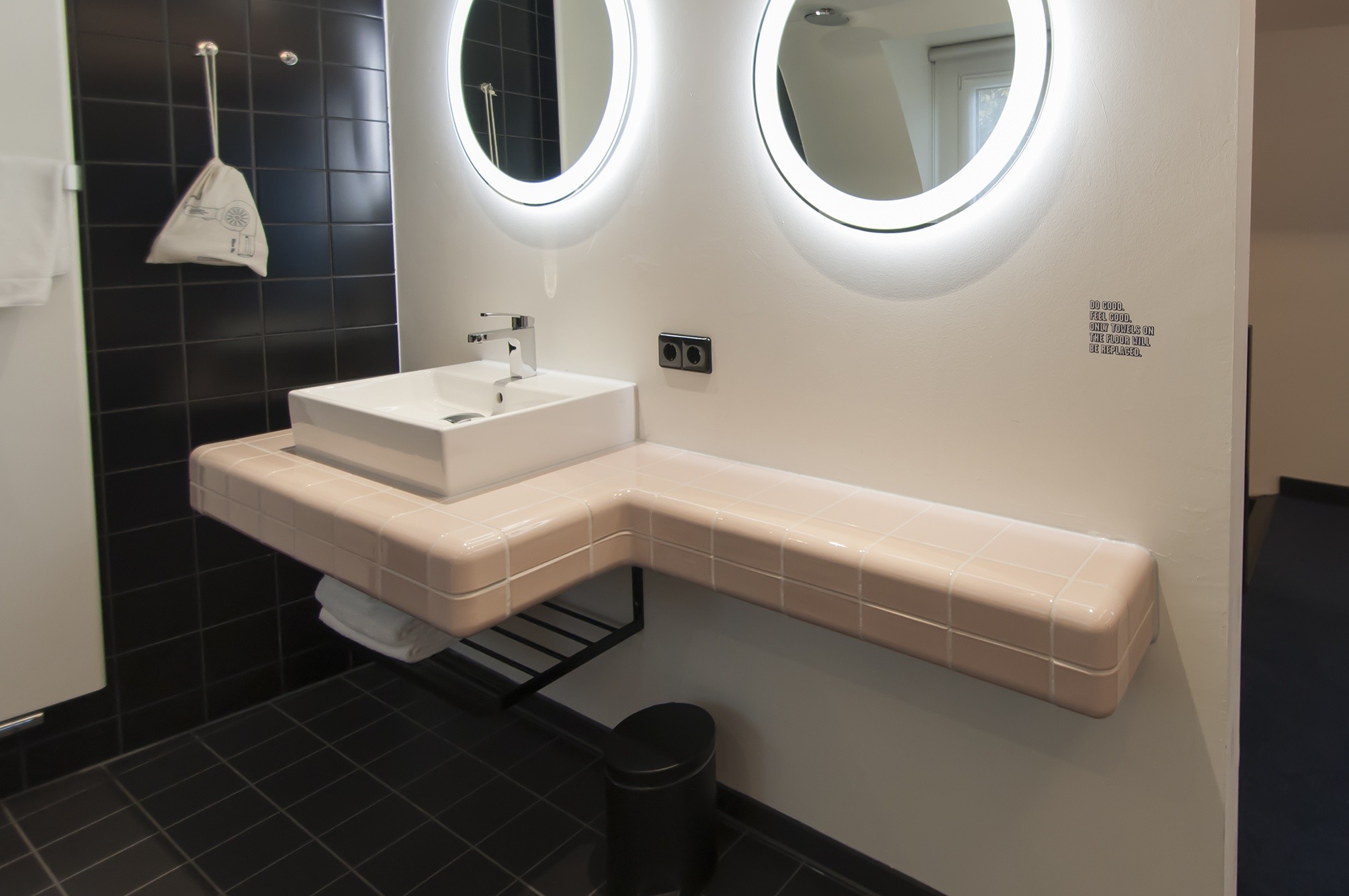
[752,0,1054,235]
[446,0,637,206]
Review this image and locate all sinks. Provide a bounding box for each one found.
[288,360,636,496]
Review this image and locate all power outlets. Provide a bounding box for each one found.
[658,333,712,374]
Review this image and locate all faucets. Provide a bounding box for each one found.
[468,313,536,379]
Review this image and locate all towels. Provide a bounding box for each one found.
[0,155,68,306]
[315,574,464,663]
